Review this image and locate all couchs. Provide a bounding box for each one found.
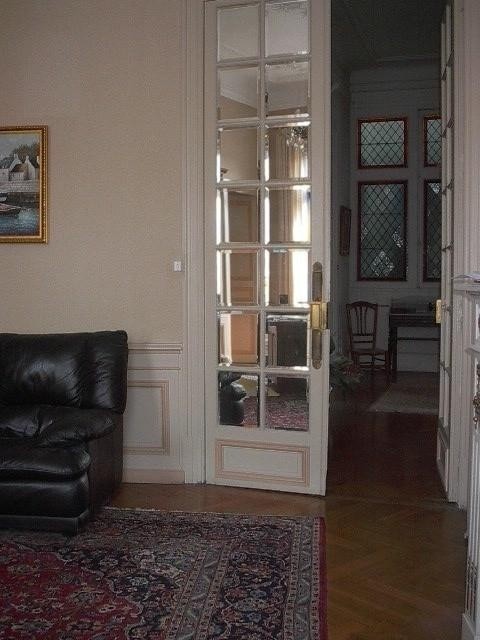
[1,329,129,537]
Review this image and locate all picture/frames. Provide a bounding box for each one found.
[338,203,352,256]
[2,124,49,245]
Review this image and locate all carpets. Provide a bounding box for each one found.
[0,506,327,640]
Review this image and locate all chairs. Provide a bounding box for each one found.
[345,300,392,385]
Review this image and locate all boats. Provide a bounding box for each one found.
[0,201,23,216]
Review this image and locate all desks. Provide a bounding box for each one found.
[388,298,444,384]
